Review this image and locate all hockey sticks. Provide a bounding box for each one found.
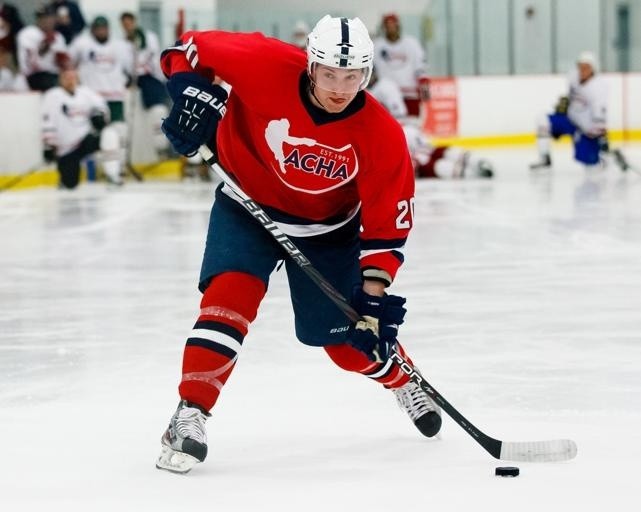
[125,27,144,182]
[195,143,578,463]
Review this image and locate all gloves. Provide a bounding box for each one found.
[345,291,409,365]
[556,96,568,116]
[160,70,229,157]
[418,76,430,100]
[595,133,608,150]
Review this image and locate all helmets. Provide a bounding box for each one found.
[306,14,375,74]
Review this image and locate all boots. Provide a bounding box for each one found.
[162,399,209,462]
[392,380,442,438]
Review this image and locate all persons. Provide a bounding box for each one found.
[530,51,610,171]
[364,64,495,179]
[290,19,314,50]
[157,15,444,474]
[372,13,431,121]
